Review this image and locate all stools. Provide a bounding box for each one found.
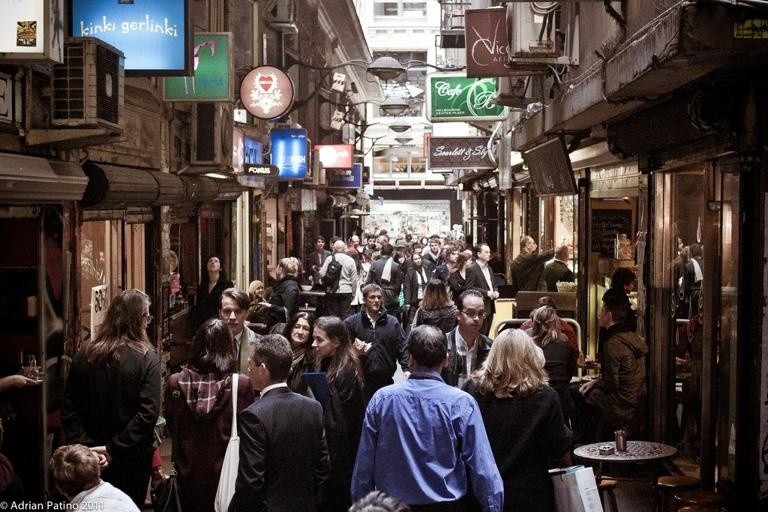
[675,489,726,505]
[655,472,699,511]
[594,473,619,511]
[677,502,726,511]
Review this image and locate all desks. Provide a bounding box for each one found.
[574,439,677,511]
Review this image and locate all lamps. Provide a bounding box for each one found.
[312,93,408,119]
[319,118,414,136]
[287,54,405,116]
[358,136,414,156]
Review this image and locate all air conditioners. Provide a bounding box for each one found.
[268,1,300,36]
[48,37,126,127]
[508,0,558,56]
[190,100,233,166]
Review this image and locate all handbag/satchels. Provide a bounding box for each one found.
[321,252,342,296]
[548,464,604,512]
[214,432,240,512]
[268,307,291,334]
[155,467,189,512]
[399,290,405,311]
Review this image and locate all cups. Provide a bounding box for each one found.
[614,431,627,452]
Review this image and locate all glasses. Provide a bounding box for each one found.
[143,315,153,324]
[459,309,487,320]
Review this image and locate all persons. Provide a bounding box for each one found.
[497,288,517,300]
[615,233,633,260]
[80,238,108,328]
[394,164,426,172]
[410,279,420,307]
[461,328,574,511]
[302,212,410,284]
[229,334,332,511]
[60,287,163,511]
[564,287,651,441]
[524,304,577,430]
[459,279,560,288]
[410,211,577,279]
[410,279,459,335]
[597,266,644,379]
[519,295,582,365]
[245,278,270,305]
[440,288,497,391]
[315,310,342,315]
[350,324,506,511]
[302,315,371,512]
[423,279,430,289]
[560,278,575,282]
[47,444,142,511]
[279,310,315,400]
[518,288,561,292]
[420,279,423,299]
[165,318,255,511]
[302,284,342,310]
[0,373,43,509]
[674,236,730,466]
[217,286,263,381]
[343,284,412,408]
[255,257,301,334]
[410,307,420,327]
[192,254,235,319]
